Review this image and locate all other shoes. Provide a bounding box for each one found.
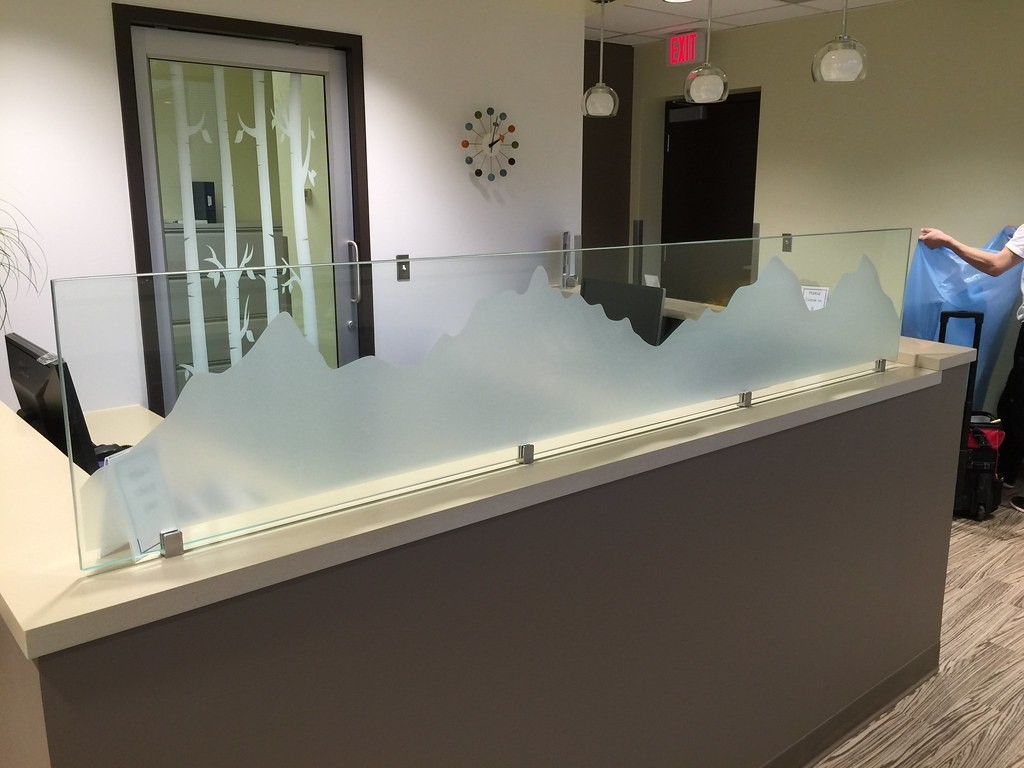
[1002,474,1016,488]
[1010,496,1024,513]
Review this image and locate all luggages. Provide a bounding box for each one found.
[938,310,1005,520]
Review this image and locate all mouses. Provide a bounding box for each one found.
[94,444,124,461]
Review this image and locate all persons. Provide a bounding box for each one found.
[917,223,1024,512]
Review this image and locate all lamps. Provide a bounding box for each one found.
[811,0,870,86]
[581,0,619,117]
[683,0,730,105]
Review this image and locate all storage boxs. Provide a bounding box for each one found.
[193,181,216,223]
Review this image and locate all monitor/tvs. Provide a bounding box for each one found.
[5,332,100,476]
[581,277,667,346]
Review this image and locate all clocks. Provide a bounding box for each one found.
[463,107,519,181]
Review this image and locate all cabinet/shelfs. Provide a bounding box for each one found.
[163,222,289,393]
